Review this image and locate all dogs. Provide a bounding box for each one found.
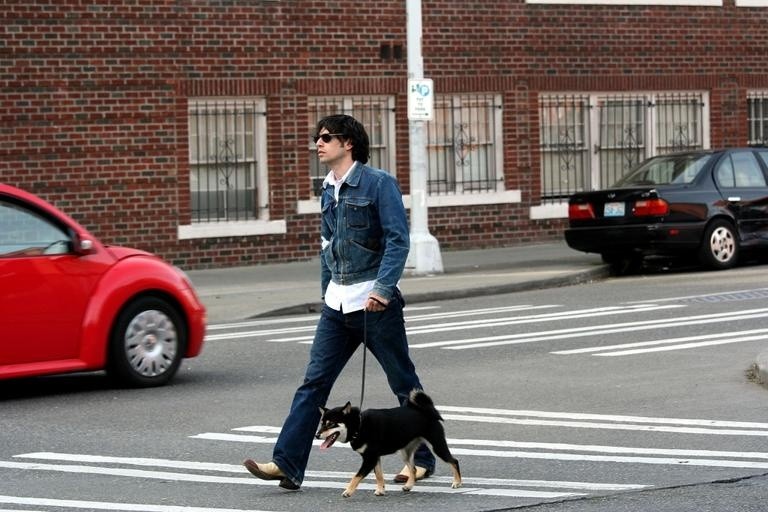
[314,388,463,499]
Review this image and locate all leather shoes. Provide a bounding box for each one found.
[243,459,299,490]
[394,464,430,483]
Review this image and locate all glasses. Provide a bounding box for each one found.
[314,132,345,143]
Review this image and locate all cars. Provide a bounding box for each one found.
[0,178,208,386]
[561,144,768,269]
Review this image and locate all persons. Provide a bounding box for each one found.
[243,114,436,489]
